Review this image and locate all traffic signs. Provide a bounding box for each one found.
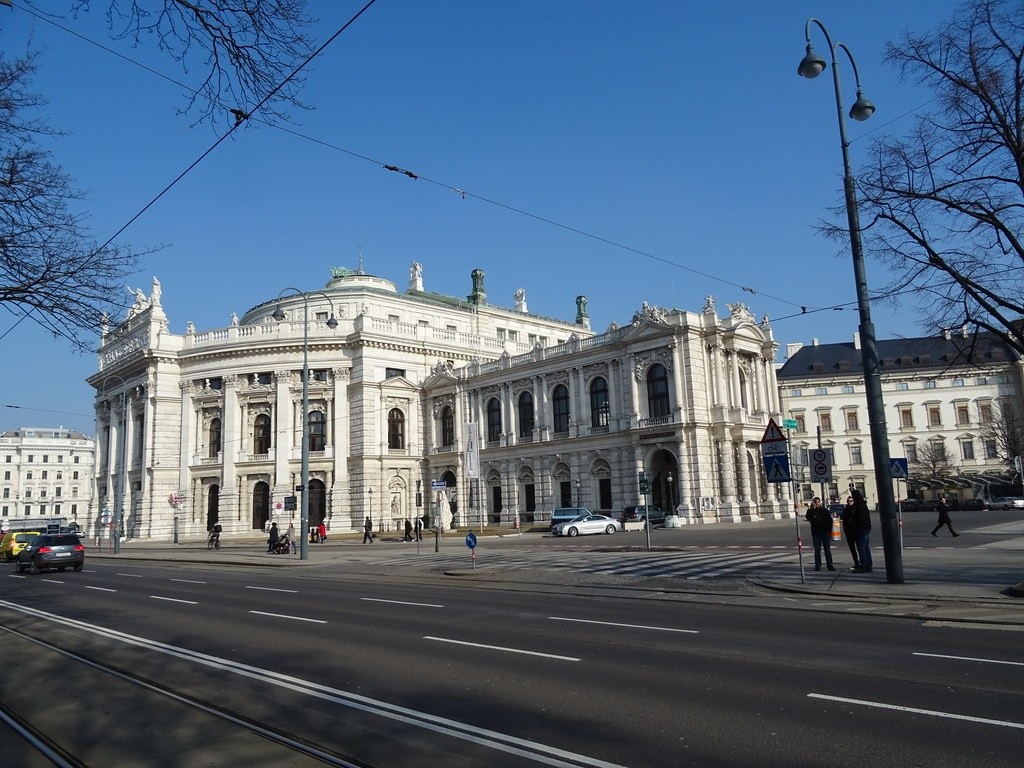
[432,481,446,489]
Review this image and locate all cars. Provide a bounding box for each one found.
[875,495,1024,512]
[826,503,847,520]
[551,514,622,537]
[71,531,86,538]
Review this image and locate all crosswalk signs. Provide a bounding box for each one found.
[763,455,792,482]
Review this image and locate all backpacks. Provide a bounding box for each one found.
[214,525,222,533]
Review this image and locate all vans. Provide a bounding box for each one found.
[552,508,594,527]
[0,531,40,563]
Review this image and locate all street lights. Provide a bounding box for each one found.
[796,18,905,587]
[273,286,338,562]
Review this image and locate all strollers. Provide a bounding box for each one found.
[273,535,291,554]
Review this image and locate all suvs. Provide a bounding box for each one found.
[623,504,671,525]
[15,531,85,575]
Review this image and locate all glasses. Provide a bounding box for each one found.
[847,499,853,502]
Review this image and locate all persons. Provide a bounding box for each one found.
[319,521,326,543]
[931,497,960,537]
[103,312,108,331]
[840,497,873,573]
[363,516,373,543]
[806,497,836,571]
[404,518,414,542]
[127,286,146,319]
[209,524,220,547]
[412,260,422,277]
[268,523,278,553]
[414,517,423,542]
[285,523,297,554]
[231,313,238,326]
[153,277,162,295]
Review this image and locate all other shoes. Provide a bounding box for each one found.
[931,532,939,537]
[850,564,872,574]
[828,567,837,571]
[403,541,406,543]
[412,539,414,542]
[815,566,820,571]
[952,534,960,537]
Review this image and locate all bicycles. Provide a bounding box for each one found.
[207,535,221,550]
